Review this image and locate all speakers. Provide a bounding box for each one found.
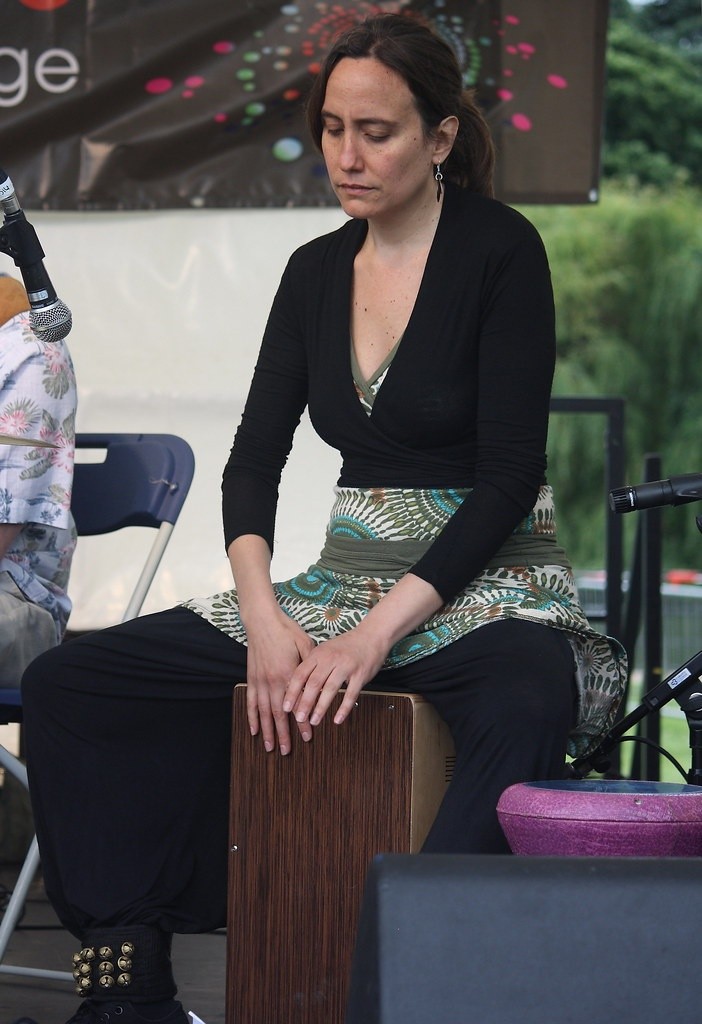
[342,851,702,1024]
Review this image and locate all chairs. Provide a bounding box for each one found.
[0,431,196,982]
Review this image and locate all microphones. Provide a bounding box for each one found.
[608,473,702,514]
[0,169,72,342]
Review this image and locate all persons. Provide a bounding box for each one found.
[21,12,627,1024]
[0,276,79,707]
